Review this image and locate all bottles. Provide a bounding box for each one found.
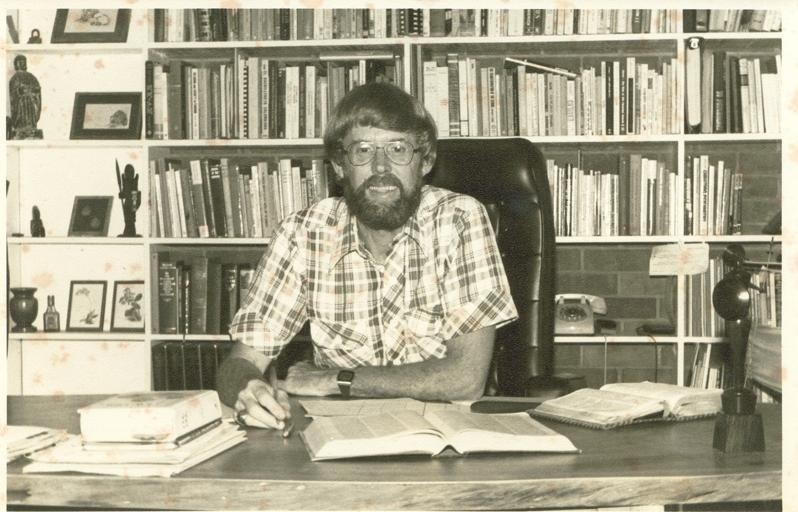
[9,286,38,333]
[43,296,61,332]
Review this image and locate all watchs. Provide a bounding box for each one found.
[334,368,357,398]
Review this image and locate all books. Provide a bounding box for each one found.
[546,153,678,236]
[297,412,585,460]
[153,342,235,389]
[8,388,250,476]
[146,157,329,238]
[686,259,777,337]
[262,341,314,386]
[145,37,779,139]
[690,343,778,402]
[533,376,730,429]
[684,153,742,235]
[151,251,255,335]
[144,8,783,44]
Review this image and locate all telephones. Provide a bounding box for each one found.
[554,293,608,335]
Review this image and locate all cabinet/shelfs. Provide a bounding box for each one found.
[146,3,781,404]
[3,40,149,341]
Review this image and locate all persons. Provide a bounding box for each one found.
[216,83,497,430]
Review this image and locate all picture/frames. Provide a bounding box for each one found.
[65,193,113,236]
[68,90,143,140]
[64,279,147,334]
[48,10,132,43]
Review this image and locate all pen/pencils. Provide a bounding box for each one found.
[269,366,277,402]
[282,423,295,438]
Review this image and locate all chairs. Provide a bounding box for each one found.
[275,135,573,398]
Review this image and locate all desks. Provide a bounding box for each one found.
[6,386,781,511]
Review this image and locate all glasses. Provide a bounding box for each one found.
[340,139,422,167]
